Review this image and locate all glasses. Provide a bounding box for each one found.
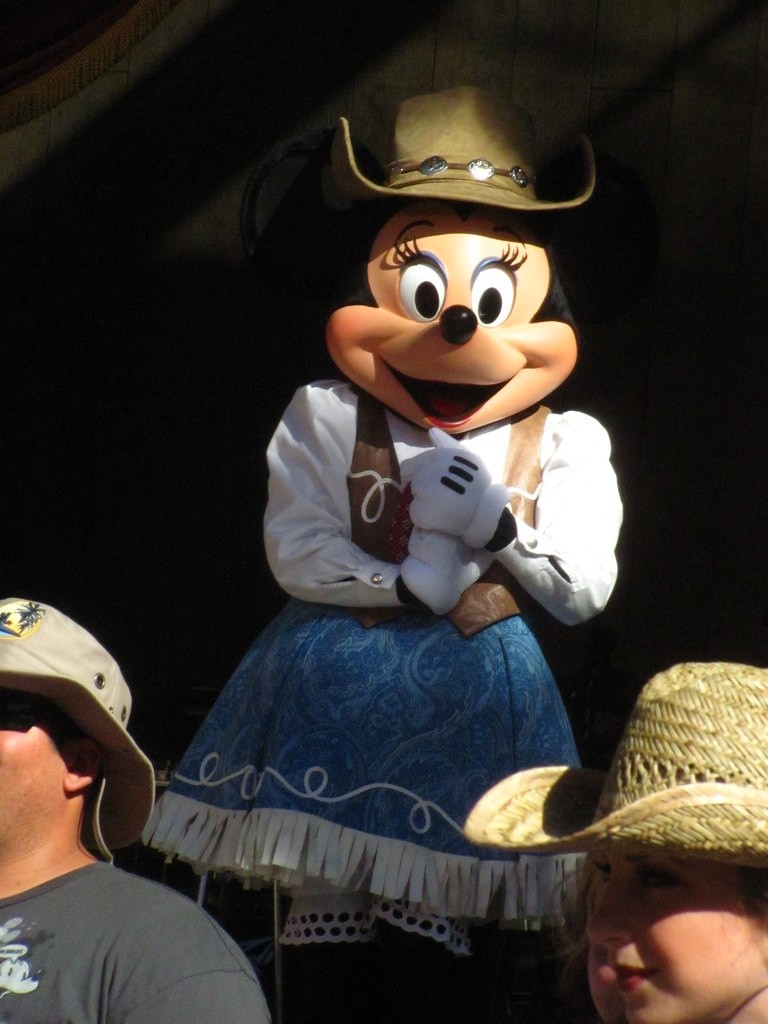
[0,699,60,731]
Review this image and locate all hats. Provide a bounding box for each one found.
[464,661,767,868]
[0,597,155,853]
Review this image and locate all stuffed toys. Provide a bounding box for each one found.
[131,78,623,1024]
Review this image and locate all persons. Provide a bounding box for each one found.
[463,665,768,1024]
[0,596,274,1024]
